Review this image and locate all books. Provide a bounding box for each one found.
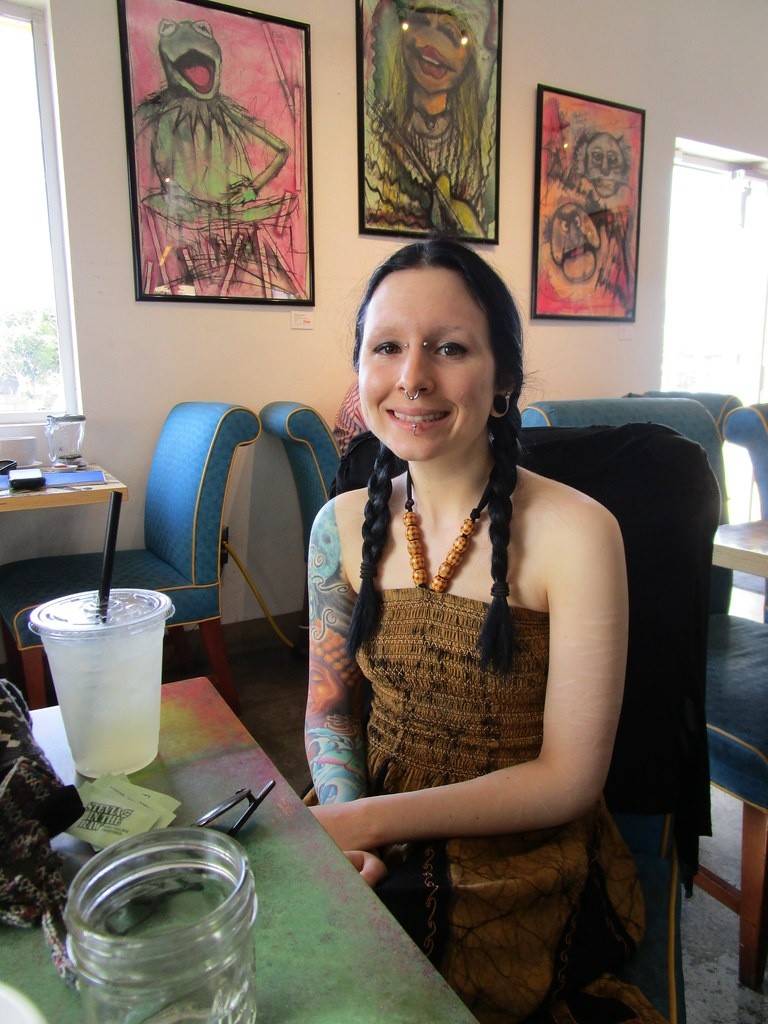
[41,471,108,488]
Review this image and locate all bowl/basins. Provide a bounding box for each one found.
[0,436,38,466]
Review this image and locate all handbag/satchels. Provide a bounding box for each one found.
[1,677,79,993]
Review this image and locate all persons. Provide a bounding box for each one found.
[304,241,668,1024]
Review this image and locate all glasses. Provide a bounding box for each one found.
[105,780,276,938]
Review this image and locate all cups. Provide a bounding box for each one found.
[65,828,258,1023]
[27,589,175,779]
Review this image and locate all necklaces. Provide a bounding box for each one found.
[404,467,489,592]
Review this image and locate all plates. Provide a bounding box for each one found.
[16,461,42,470]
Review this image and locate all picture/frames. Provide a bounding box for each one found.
[356,0,503,244]
[529,84,646,323]
[117,0,314,307]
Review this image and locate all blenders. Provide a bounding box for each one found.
[47,413,88,469]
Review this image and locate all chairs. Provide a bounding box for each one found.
[333,422,721,1024]
[259,392,768,988]
[0,402,261,715]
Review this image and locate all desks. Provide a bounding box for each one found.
[712,518,768,578]
[0,465,130,512]
[0,678,480,1024]
